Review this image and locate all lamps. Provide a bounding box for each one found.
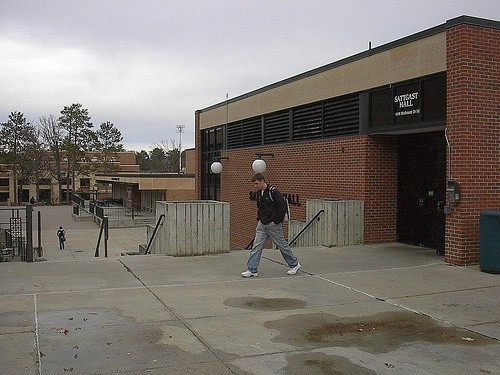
[210,157,229,174]
[250,152,274,174]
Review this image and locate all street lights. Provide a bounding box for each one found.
[176,124,186,173]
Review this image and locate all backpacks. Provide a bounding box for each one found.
[59,230,63,236]
[258,186,290,222]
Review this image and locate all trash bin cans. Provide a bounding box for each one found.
[479,210,500,274]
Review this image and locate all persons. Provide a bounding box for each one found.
[57,226,66,249]
[30,195,35,211]
[241,173,301,278]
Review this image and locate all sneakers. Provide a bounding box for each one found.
[287,263,301,274]
[241,270,258,278]
